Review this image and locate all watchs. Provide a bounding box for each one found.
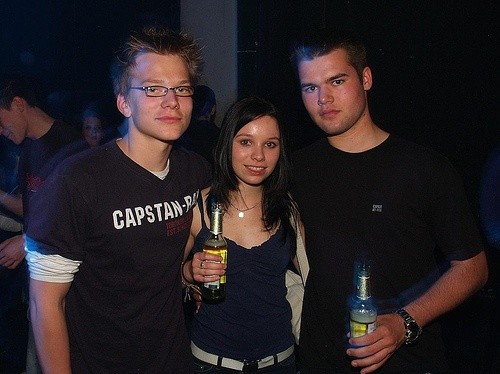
[392,306,423,345]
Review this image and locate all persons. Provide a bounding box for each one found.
[0,71,222,373]
[24,23,212,374]
[290,26,488,374]
[179,95,306,374]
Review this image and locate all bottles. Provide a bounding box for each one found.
[348,264,377,348]
[202,201,227,303]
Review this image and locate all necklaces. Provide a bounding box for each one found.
[230,203,259,217]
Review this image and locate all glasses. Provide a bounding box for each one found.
[129,85,195,97]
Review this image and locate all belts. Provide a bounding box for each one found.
[191,339,294,373]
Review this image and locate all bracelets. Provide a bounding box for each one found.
[182,261,192,283]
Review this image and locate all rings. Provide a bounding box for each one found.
[200,260,206,267]
